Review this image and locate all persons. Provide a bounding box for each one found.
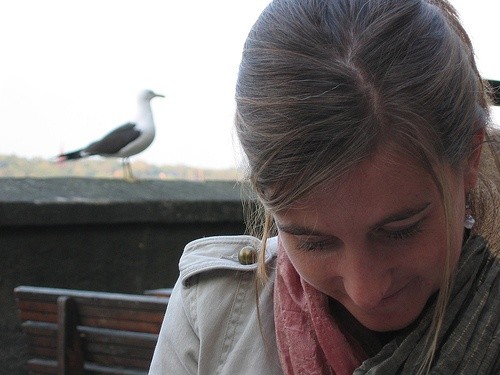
[144,1,499,374]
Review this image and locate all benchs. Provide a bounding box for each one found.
[15,285,170,375]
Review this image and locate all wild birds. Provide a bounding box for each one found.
[53,90,166,180]
